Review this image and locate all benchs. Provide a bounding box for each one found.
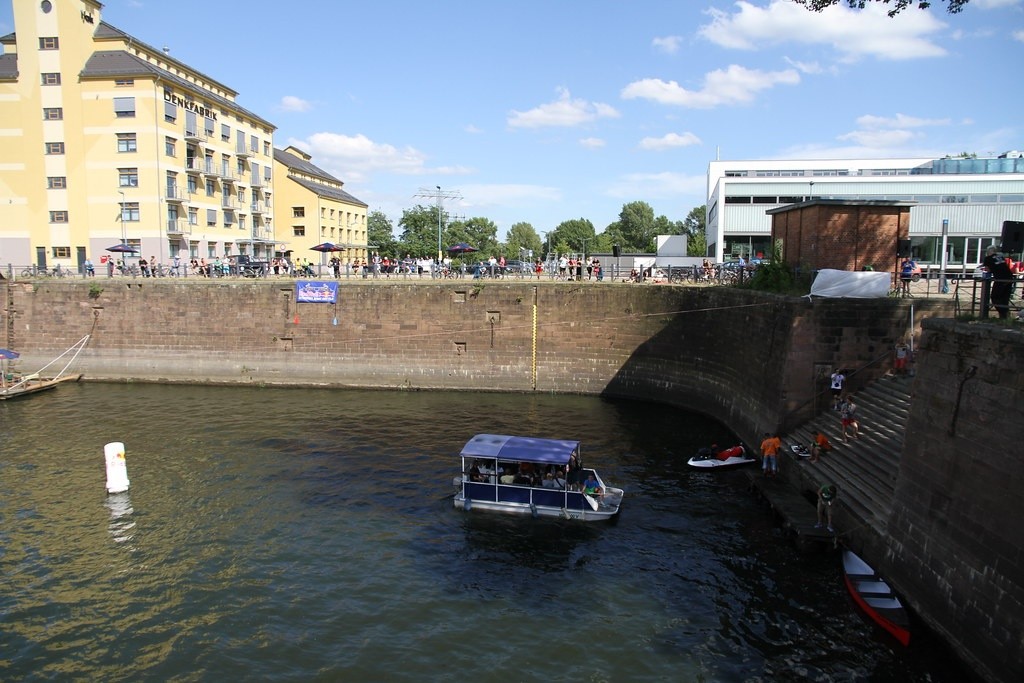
[858,585,896,600]
[848,568,887,583]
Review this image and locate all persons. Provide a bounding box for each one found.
[984,246,1013,320]
[901,256,915,298]
[737,249,768,285]
[84,258,94,276]
[760,432,780,481]
[702,259,718,285]
[272,257,313,278]
[138,255,237,278]
[831,368,845,410]
[108,255,126,278]
[470,459,601,499]
[814,484,837,533]
[893,337,911,379]
[839,396,859,443]
[353,251,506,279]
[330,255,341,279]
[535,257,543,279]
[623,269,664,284]
[808,430,831,464]
[558,254,603,282]
[710,441,746,460]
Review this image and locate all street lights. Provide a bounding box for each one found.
[118,189,128,268]
[541,230,560,261]
[437,185,442,266]
[810,181,815,201]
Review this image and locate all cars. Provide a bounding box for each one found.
[721,261,740,270]
[465,262,504,274]
[504,260,536,273]
[367,260,423,274]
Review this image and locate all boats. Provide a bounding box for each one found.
[839,547,912,647]
[454,434,626,522]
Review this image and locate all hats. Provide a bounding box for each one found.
[547,473,552,477]
[557,471,563,475]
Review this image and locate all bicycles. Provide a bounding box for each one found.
[243,265,264,278]
[507,263,533,280]
[671,268,700,286]
[436,268,462,280]
[707,267,753,286]
[486,265,504,280]
[20,264,179,279]
[549,264,567,281]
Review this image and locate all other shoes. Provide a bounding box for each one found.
[827,526,834,532]
[600,501,607,508]
[814,524,823,528]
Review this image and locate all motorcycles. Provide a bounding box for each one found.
[688,446,756,472]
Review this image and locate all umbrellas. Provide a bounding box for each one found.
[0,349,20,386]
[105,244,139,261]
[310,243,345,253]
[446,242,479,279]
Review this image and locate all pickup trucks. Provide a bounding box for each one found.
[198,254,270,276]
